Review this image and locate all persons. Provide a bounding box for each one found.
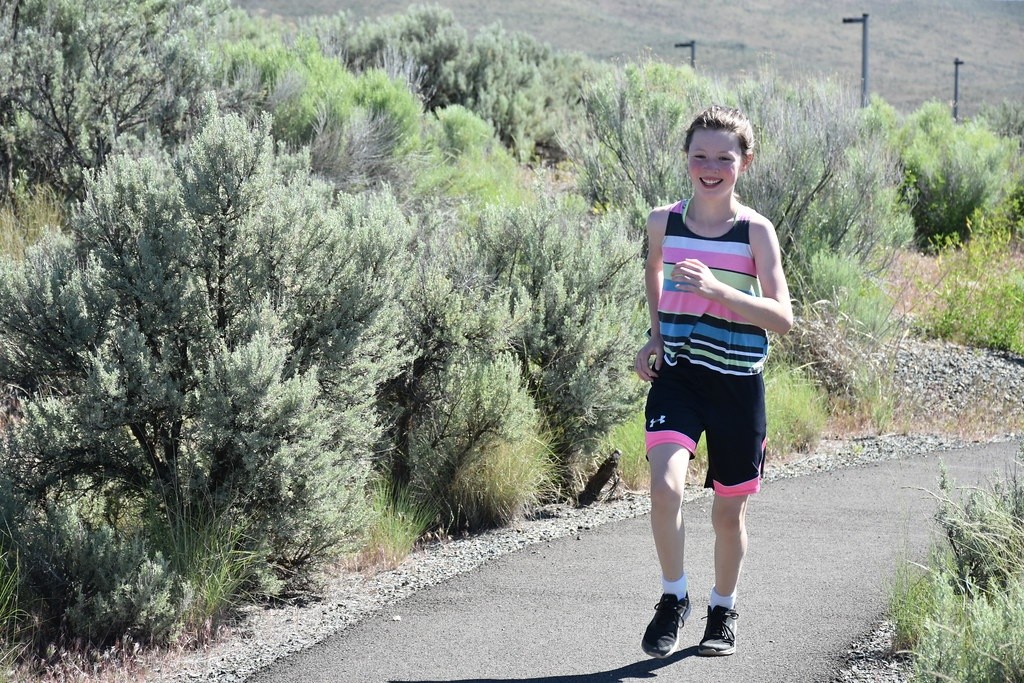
[634,108,793,658]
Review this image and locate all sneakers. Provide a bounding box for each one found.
[697,605,739,655]
[641,590,692,659]
